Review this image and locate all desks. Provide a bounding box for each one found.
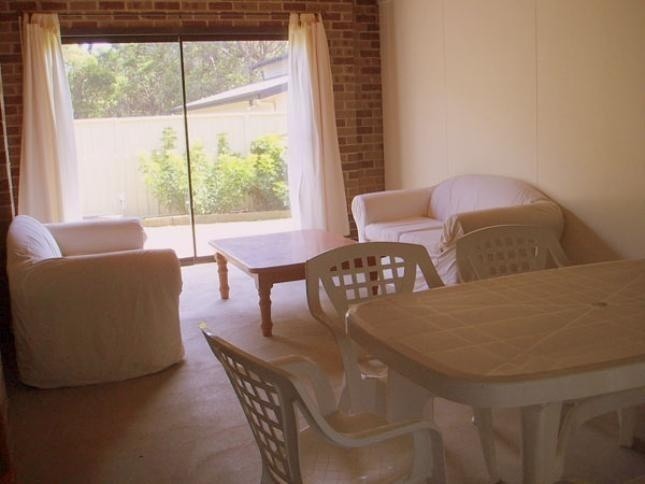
[345,253,644,483]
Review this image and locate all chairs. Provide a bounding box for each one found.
[306,226,568,416]
[350,173,565,295]
[199,327,447,484]
[4,214,185,389]
